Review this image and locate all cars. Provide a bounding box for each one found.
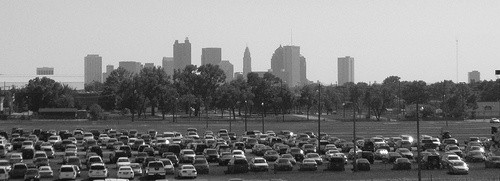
[490,117,500,123]
[0,128,500,181]
